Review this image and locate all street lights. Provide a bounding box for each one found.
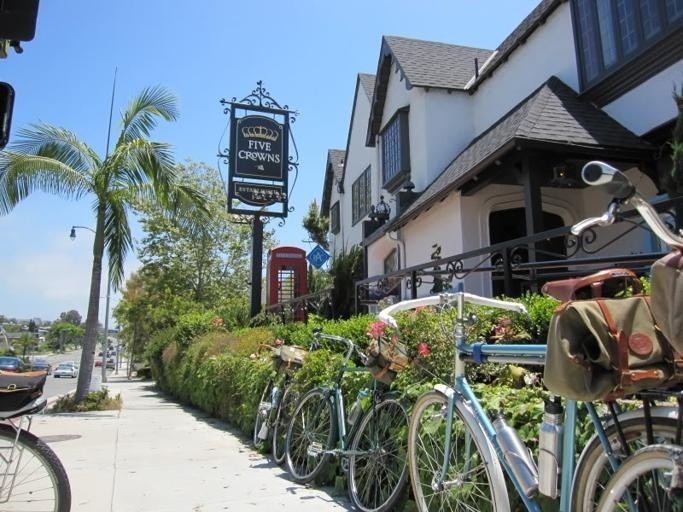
[69,225,112,383]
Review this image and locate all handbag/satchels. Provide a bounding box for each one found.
[271,346,306,371]
[543,295,681,401]
[365,336,408,386]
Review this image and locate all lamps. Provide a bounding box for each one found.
[375,195,398,226]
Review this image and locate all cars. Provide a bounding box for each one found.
[53,360,78,378]
[94,345,120,370]
[30,358,52,375]
[0,357,30,373]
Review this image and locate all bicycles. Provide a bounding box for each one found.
[253,338,313,466]
[571,161,682,511]
[377,283,682,511]
[284,327,418,511]
[0,357,70,511]
